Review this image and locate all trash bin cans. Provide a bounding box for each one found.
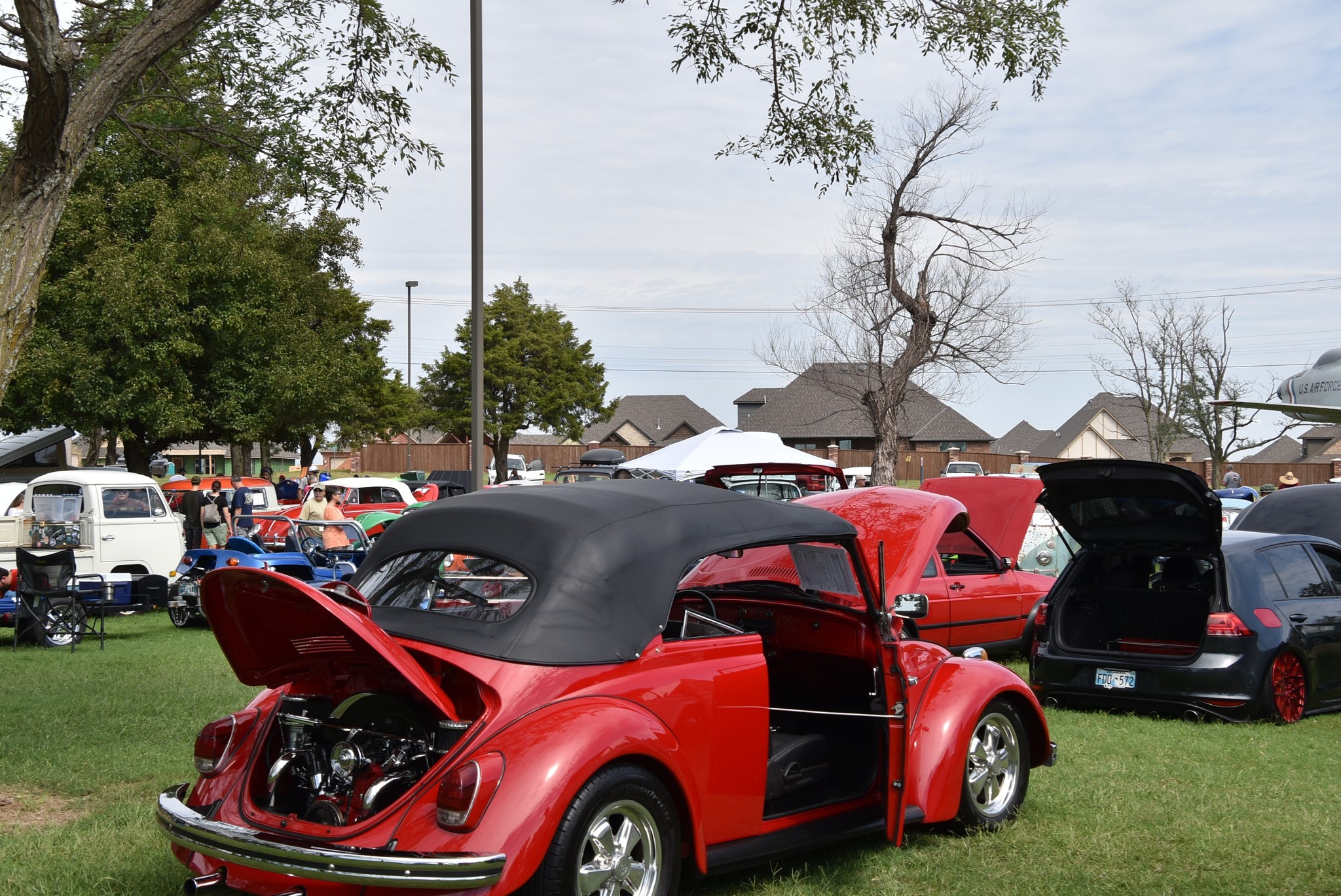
[320,474,330,482]
[166,462,175,475]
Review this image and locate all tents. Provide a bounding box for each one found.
[619,425,836,492]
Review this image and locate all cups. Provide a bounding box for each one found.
[102,582,116,600]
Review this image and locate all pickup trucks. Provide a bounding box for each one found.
[157,476,306,550]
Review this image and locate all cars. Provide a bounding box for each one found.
[115,451,169,479]
[152,480,1057,895]
[168,478,537,628]
[685,457,1341,725]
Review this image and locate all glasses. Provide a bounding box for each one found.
[312,471,319,474]
[118,490,127,494]
[335,492,341,495]
[314,489,323,492]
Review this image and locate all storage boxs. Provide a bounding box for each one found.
[32,494,84,522]
[30,520,82,550]
[78,571,132,605]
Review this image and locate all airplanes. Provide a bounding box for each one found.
[1206,349,1341,423]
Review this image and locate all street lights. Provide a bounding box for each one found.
[405,281,418,473]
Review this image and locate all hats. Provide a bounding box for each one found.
[1259,484,1276,492]
[313,483,326,490]
[309,466,320,472]
[855,474,868,480]
[1279,472,1299,485]
[279,475,285,479]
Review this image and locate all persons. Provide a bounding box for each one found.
[1220,464,1241,489]
[0,567,52,598]
[258,467,276,487]
[854,473,868,488]
[279,475,285,483]
[230,474,254,538]
[612,468,633,479]
[169,467,187,483]
[179,476,205,549]
[1277,473,1301,490]
[1259,484,1275,498]
[321,486,354,551]
[105,489,147,512]
[508,469,523,481]
[298,466,319,500]
[301,483,328,549]
[7,491,26,516]
[200,480,232,550]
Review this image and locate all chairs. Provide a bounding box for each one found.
[283,535,318,568]
[1199,567,1225,631]
[349,496,360,504]
[660,618,833,814]
[12,547,121,654]
[1147,578,1206,644]
[251,533,274,554]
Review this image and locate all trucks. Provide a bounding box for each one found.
[0,470,188,622]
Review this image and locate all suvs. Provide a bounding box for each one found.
[550,448,625,483]
[485,454,545,480]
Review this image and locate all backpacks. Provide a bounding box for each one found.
[204,495,221,523]
